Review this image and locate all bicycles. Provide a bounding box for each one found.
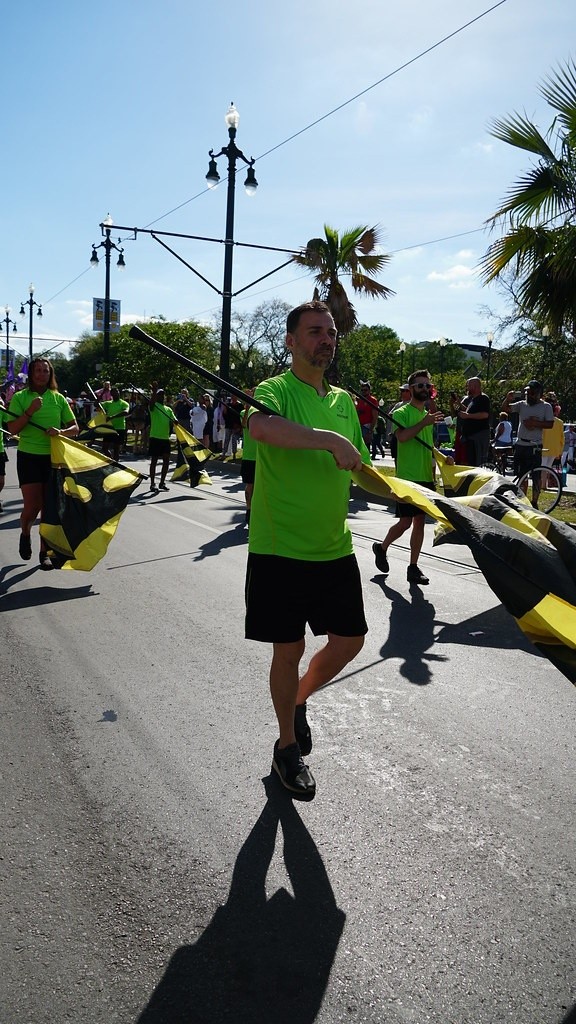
[477,437,563,515]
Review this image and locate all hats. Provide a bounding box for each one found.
[398,384,410,391]
[523,380,541,390]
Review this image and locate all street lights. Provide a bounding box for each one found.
[203,101,259,395]
[0,303,19,373]
[19,283,44,361]
[439,335,447,408]
[541,324,550,388]
[88,212,127,362]
[399,341,407,385]
[486,331,495,382]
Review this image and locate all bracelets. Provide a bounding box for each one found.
[25,410,32,418]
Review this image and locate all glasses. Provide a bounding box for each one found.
[411,382,432,389]
[361,388,369,391]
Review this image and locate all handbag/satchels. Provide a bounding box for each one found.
[548,458,567,487]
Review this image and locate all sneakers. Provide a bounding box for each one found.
[273,737,317,793]
[408,566,429,583]
[373,542,389,573]
[295,701,313,757]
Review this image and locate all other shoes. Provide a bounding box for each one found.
[19,533,31,560]
[39,552,53,569]
[160,484,169,490]
[151,486,158,492]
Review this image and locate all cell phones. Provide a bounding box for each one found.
[450,392,457,403]
[513,392,522,398]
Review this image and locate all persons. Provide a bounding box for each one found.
[0,378,27,513]
[245,302,374,794]
[372,368,454,585]
[7,359,79,570]
[347,377,490,469]
[61,382,258,524]
[490,380,576,510]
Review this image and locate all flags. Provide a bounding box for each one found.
[74,410,118,444]
[173,422,212,488]
[169,437,190,483]
[352,447,576,689]
[39,434,144,571]
